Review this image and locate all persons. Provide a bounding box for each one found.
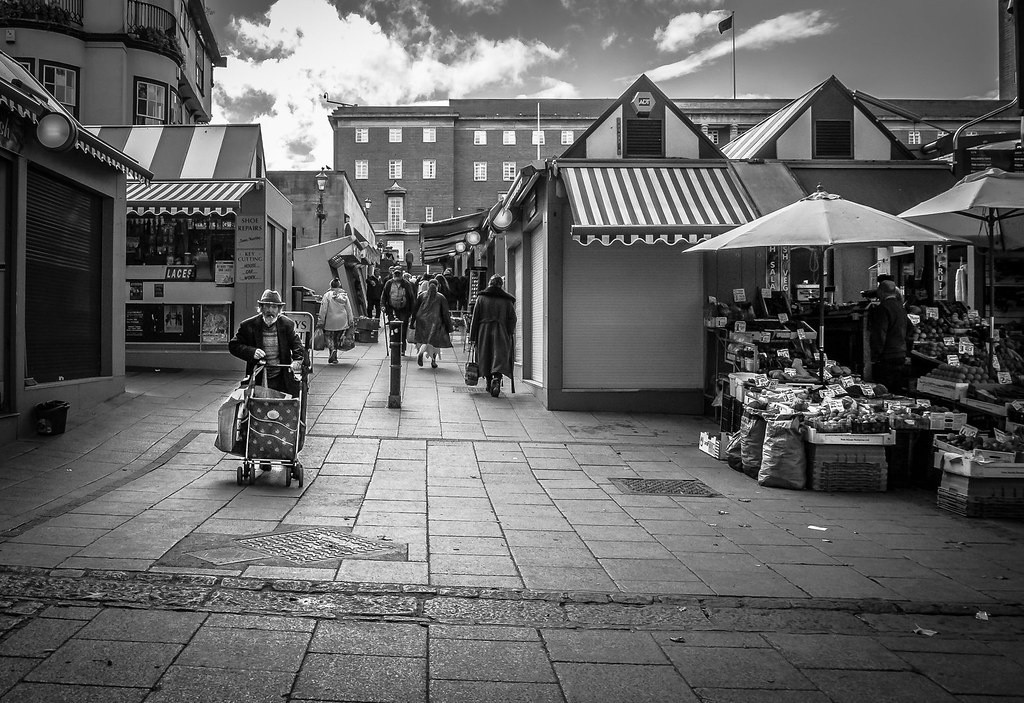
[863,274,908,394]
[469,274,518,397]
[379,270,416,355]
[384,264,470,331]
[228,289,305,472]
[405,248,414,273]
[410,279,454,369]
[366,275,380,318]
[314,278,355,365]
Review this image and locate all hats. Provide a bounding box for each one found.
[394,270,401,275]
[257,289,286,305]
[879,280,895,292]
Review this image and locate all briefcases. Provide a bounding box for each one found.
[406,326,415,343]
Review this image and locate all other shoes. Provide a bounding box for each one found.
[260,459,271,471]
[491,378,500,397]
[328,350,338,364]
[431,363,438,368]
[401,352,405,356]
[418,354,424,367]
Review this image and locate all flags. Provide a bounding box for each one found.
[719,16,732,34]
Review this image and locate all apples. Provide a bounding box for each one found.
[913,317,1024,385]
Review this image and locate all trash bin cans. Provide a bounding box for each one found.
[34,400,71,436]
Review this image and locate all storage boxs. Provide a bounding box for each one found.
[790,320,817,340]
[728,372,761,402]
[353,317,380,331]
[765,325,791,340]
[804,424,896,446]
[703,317,728,327]
[750,331,771,343]
[927,412,968,431]
[354,328,378,343]
[759,288,793,318]
[933,448,1024,478]
[698,430,736,460]
[917,376,969,400]
[795,284,821,301]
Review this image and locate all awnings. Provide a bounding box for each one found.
[420,212,486,264]
[126,178,258,217]
[554,162,766,246]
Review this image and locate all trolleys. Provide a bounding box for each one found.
[236,365,308,488]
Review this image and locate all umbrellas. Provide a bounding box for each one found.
[895,168,1024,381]
[682,185,974,388]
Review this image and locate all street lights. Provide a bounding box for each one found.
[364,197,373,216]
[378,239,384,252]
[315,167,329,244]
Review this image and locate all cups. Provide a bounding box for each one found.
[184,253,191,264]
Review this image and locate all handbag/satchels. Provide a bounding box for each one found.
[338,324,356,351]
[428,322,454,348]
[309,328,325,351]
[464,343,479,386]
[213,362,266,452]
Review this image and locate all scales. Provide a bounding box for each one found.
[853,290,880,311]
